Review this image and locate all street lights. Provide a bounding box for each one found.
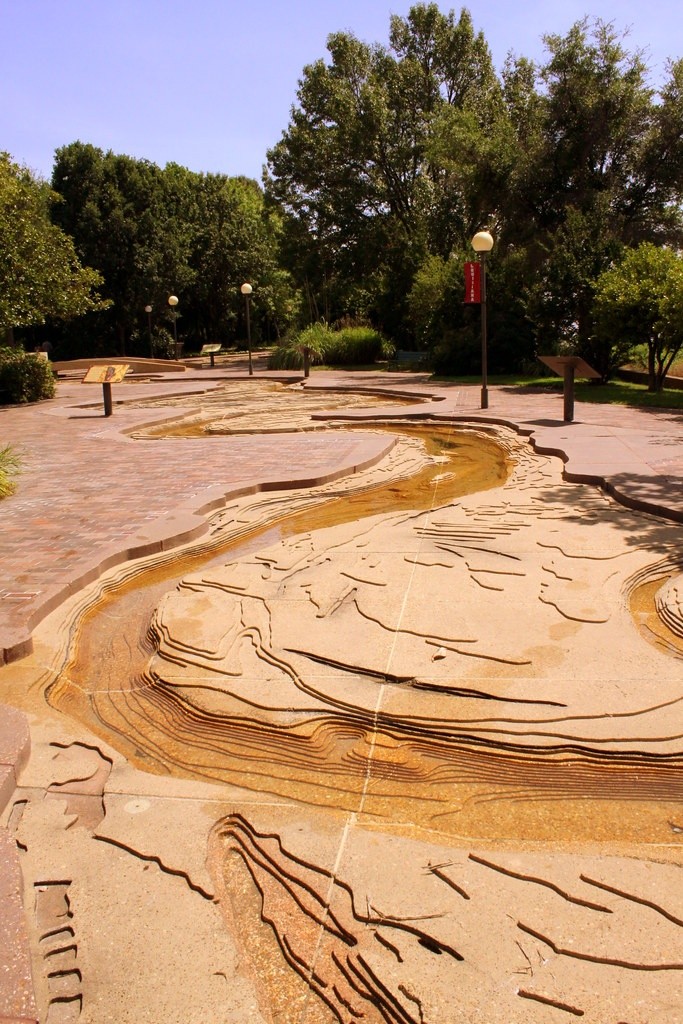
[145,306,154,359]
[168,296,179,362]
[471,232,493,409]
[240,283,254,376]
